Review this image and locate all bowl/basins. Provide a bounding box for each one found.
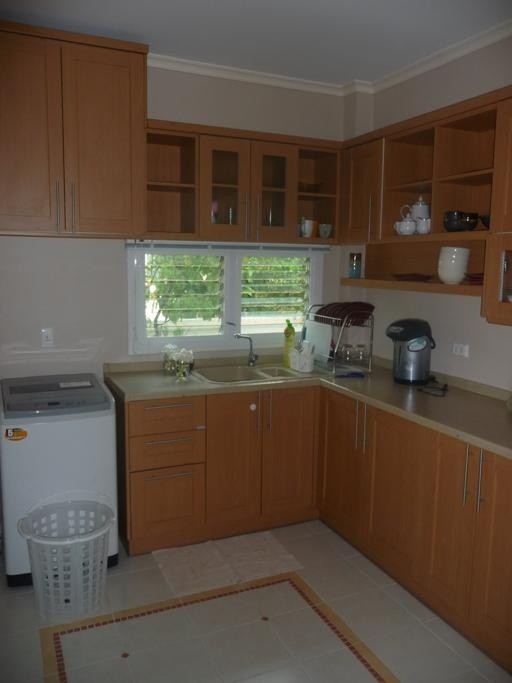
[444,208,489,232]
[437,246,470,286]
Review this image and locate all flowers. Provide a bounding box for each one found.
[159,341,195,384]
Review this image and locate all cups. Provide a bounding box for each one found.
[341,344,354,361]
[159,350,176,373]
[353,344,367,361]
[175,360,190,384]
[300,219,333,239]
[210,201,219,224]
[349,252,362,278]
[223,207,234,225]
[289,351,314,372]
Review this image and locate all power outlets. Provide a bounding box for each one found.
[453,342,472,358]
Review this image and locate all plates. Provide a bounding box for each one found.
[314,301,375,327]
[463,271,483,285]
[393,272,433,281]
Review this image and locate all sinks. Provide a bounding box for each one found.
[254,363,313,381]
[190,365,268,385]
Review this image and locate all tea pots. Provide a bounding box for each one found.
[394,196,431,236]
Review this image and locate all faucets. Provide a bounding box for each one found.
[233,333,259,367]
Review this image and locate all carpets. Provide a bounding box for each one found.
[38,569,405,683]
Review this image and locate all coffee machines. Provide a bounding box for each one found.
[385,317,435,386]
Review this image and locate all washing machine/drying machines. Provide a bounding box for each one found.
[0,372,120,589]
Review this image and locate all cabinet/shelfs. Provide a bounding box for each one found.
[484,233,512,324]
[199,134,296,243]
[383,105,497,238]
[149,129,200,240]
[491,98,512,230]
[207,380,320,542]
[319,380,435,615]
[0,18,148,241]
[339,138,382,244]
[436,429,512,678]
[102,374,207,557]
[295,144,339,243]
[339,238,489,295]
[298,302,375,378]
